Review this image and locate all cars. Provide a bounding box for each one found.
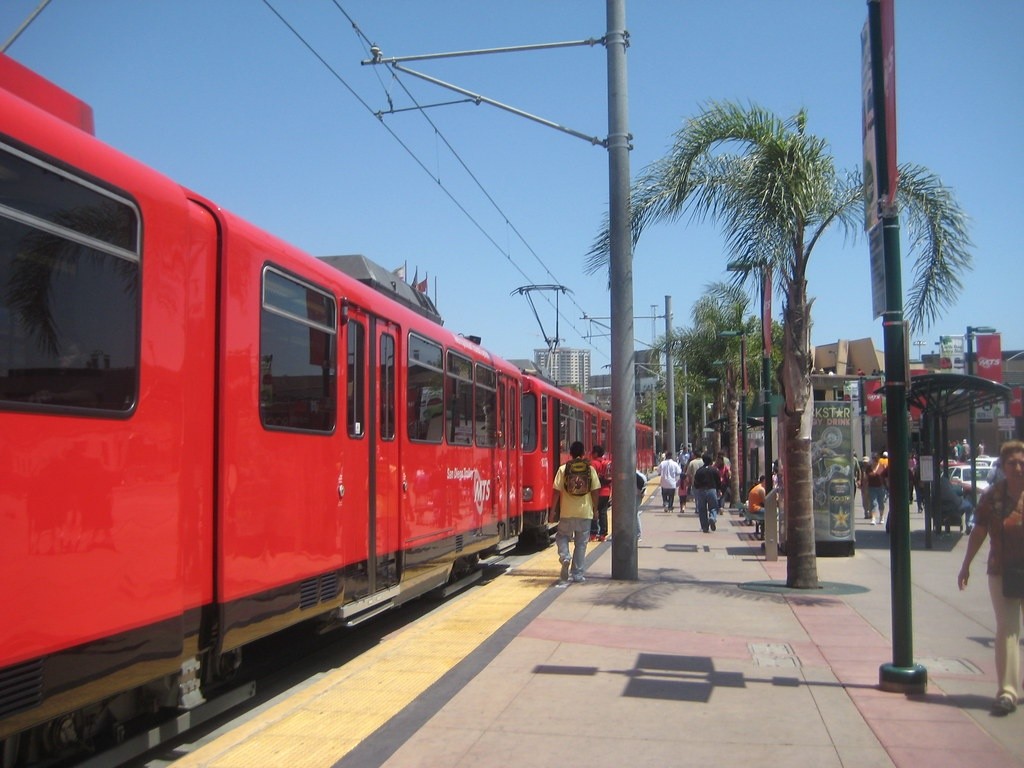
[940,454,999,504]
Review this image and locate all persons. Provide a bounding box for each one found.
[589,438,985,544]
[547,441,601,582]
[956,441,1024,714]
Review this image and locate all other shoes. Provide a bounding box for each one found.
[637,535,642,541]
[992,696,1014,714]
[870,518,876,525]
[560,558,570,580]
[669,509,673,514]
[703,527,708,533]
[709,518,716,531]
[598,534,606,542]
[664,505,668,512]
[879,515,885,523]
[719,507,724,515]
[589,533,597,542]
[864,515,872,519]
[680,508,685,513]
[573,574,585,582]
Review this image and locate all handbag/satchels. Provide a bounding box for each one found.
[1002,568,1024,599]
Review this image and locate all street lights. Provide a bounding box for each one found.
[717,330,749,509]
[713,359,734,404]
[725,256,775,555]
[913,340,927,360]
[706,377,723,460]
[859,375,872,455]
[832,386,839,400]
[965,324,996,517]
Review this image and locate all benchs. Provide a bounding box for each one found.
[742,510,765,541]
[735,503,755,526]
[922,497,964,534]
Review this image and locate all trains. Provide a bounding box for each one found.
[1,50,656,768]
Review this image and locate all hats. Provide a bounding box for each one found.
[862,456,870,462]
[882,451,888,457]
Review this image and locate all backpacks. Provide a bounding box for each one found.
[594,458,612,484]
[564,458,592,496]
[636,471,647,489]
